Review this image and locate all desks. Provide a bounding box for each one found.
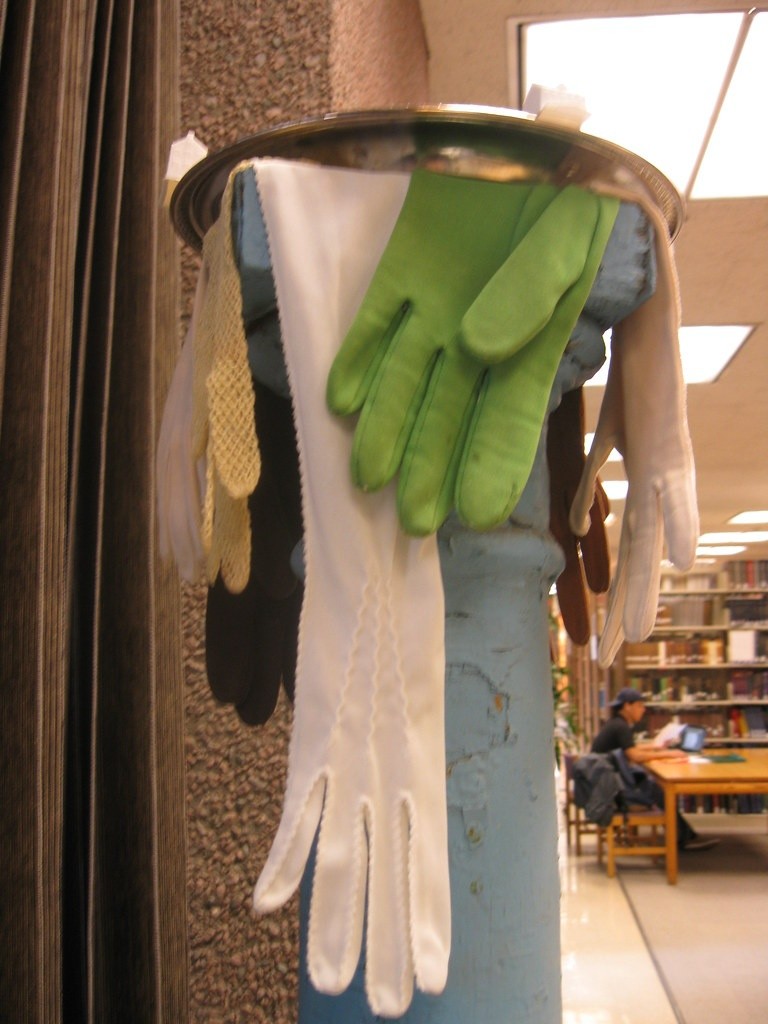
[643,746,768,884]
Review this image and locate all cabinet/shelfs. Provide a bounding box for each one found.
[566,583,768,755]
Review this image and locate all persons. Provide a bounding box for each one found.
[589,688,722,850]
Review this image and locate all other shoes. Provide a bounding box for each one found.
[684,838,721,850]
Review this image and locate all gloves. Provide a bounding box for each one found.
[154,158,261,594]
[547,385,611,644]
[206,375,306,733]
[253,156,450,1018]
[569,184,700,667]
[326,169,620,537]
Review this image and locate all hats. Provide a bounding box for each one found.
[608,688,650,709]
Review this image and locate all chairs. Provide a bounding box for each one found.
[576,755,665,876]
[563,753,635,854]
